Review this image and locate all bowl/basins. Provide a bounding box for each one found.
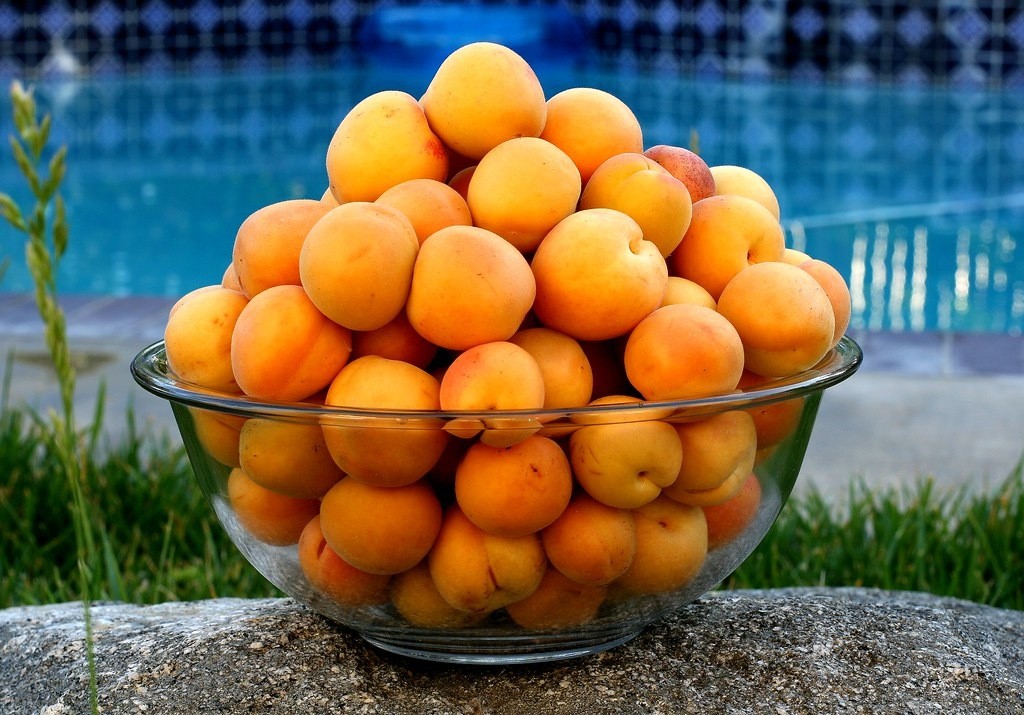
[128,336,863,666]
